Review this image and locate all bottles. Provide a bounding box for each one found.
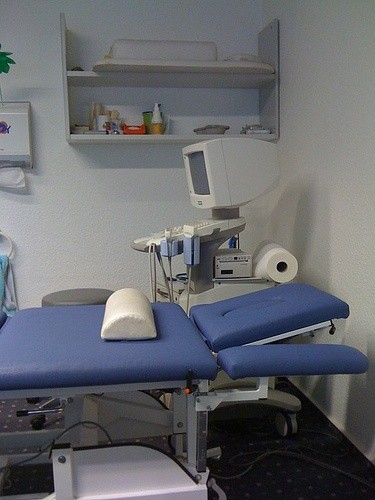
[151,102,163,134]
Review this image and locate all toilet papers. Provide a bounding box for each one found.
[253,242,298,283]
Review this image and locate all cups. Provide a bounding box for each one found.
[144,113,162,133]
[97,115,108,131]
[110,120,119,130]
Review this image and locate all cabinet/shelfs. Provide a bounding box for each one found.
[57,11,281,144]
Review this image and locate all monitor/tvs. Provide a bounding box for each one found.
[182,138,280,209]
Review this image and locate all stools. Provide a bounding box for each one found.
[30,288,115,429]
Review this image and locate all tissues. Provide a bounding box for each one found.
[0,102,34,190]
